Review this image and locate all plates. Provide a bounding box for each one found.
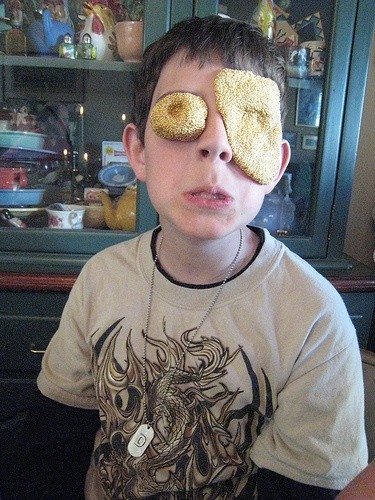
[0,208,46,218]
[97,164,137,195]
[0,146,58,158]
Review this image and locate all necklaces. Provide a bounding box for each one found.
[127,224,242,458]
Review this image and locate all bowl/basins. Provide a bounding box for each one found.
[76,205,114,227]
[0,189,47,205]
[0,130,48,150]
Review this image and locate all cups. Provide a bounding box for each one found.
[45,205,86,229]
[115,21,144,62]
[84,188,109,202]
[0,168,28,189]
[283,46,310,79]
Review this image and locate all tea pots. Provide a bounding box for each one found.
[97,184,137,231]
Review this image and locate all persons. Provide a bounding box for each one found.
[18,15,368,500]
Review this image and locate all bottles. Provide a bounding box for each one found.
[5,20,26,56]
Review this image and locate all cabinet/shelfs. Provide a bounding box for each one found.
[0,0,375,270]
[0,254,375,499]
